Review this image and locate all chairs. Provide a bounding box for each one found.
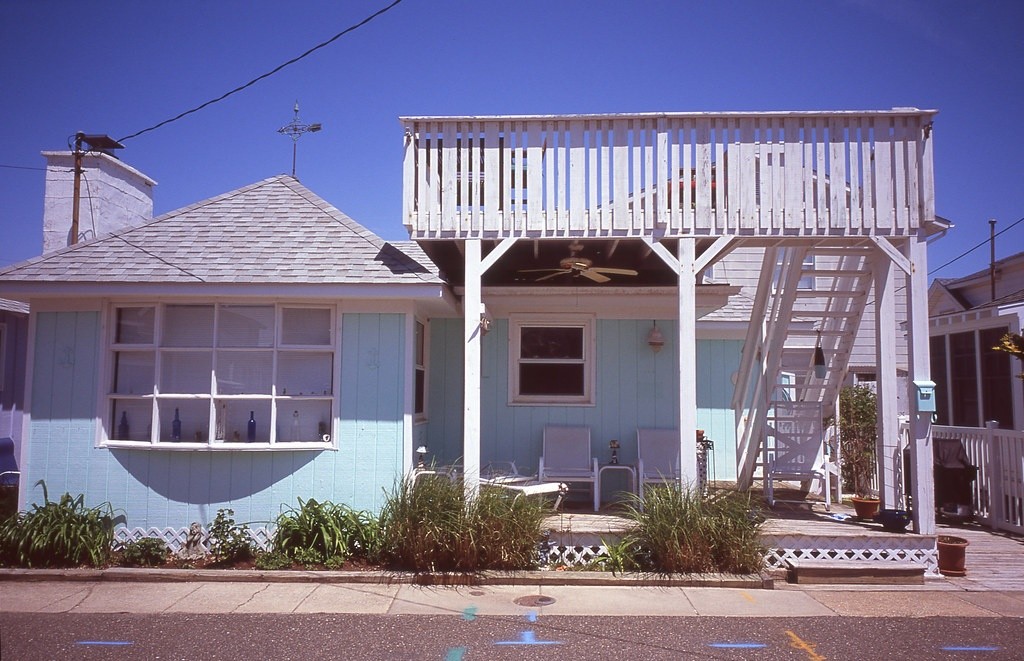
[453,460,569,513]
[768,400,831,512]
[538,425,598,512]
[636,427,681,512]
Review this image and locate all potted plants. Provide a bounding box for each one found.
[837,386,880,518]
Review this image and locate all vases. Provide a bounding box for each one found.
[937,534,970,576]
[880,508,911,532]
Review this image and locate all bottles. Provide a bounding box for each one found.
[291,411,300,441]
[171,408,181,438]
[319,422,326,440]
[247,411,256,442]
[118,411,128,440]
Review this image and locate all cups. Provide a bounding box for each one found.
[194,431,201,441]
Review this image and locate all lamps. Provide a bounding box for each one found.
[647,320,665,352]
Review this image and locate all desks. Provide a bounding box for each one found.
[410,467,457,501]
[598,464,635,511]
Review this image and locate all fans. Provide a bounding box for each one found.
[516,239,638,282]
